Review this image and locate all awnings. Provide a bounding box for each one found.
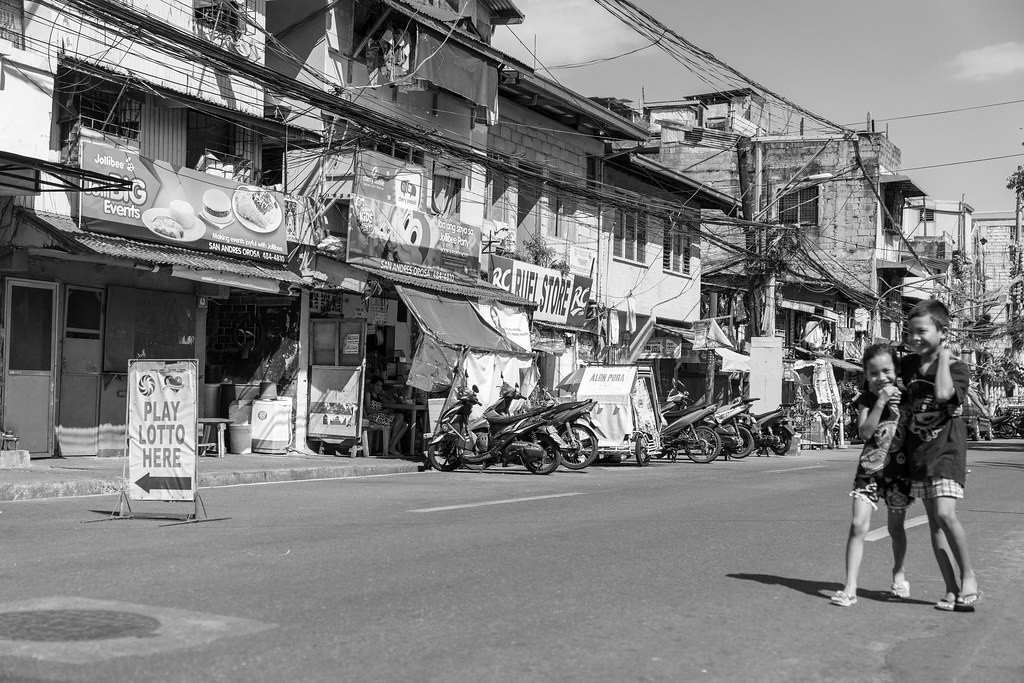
[393,283,537,357]
[0,150,133,191]
[19,212,312,287]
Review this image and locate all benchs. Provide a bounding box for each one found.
[317,420,370,457]
[198,418,233,457]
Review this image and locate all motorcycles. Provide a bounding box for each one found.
[989,409,1024,440]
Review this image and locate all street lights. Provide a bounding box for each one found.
[740,172,834,339]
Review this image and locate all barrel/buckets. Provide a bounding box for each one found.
[230,424,253,454]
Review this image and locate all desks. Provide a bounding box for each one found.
[385,403,427,455]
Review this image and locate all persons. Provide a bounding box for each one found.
[365,375,408,457]
[830,299,982,610]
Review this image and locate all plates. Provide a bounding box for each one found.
[197,211,235,229]
[141,208,206,241]
[232,186,282,233]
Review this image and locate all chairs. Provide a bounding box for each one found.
[363,405,401,456]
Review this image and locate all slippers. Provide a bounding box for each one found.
[891,581,910,598]
[832,591,858,607]
[957,591,982,607]
[935,597,957,611]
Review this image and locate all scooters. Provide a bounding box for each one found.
[425,370,572,475]
[659,377,800,464]
[480,369,609,470]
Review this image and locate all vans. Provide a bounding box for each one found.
[960,390,993,441]
